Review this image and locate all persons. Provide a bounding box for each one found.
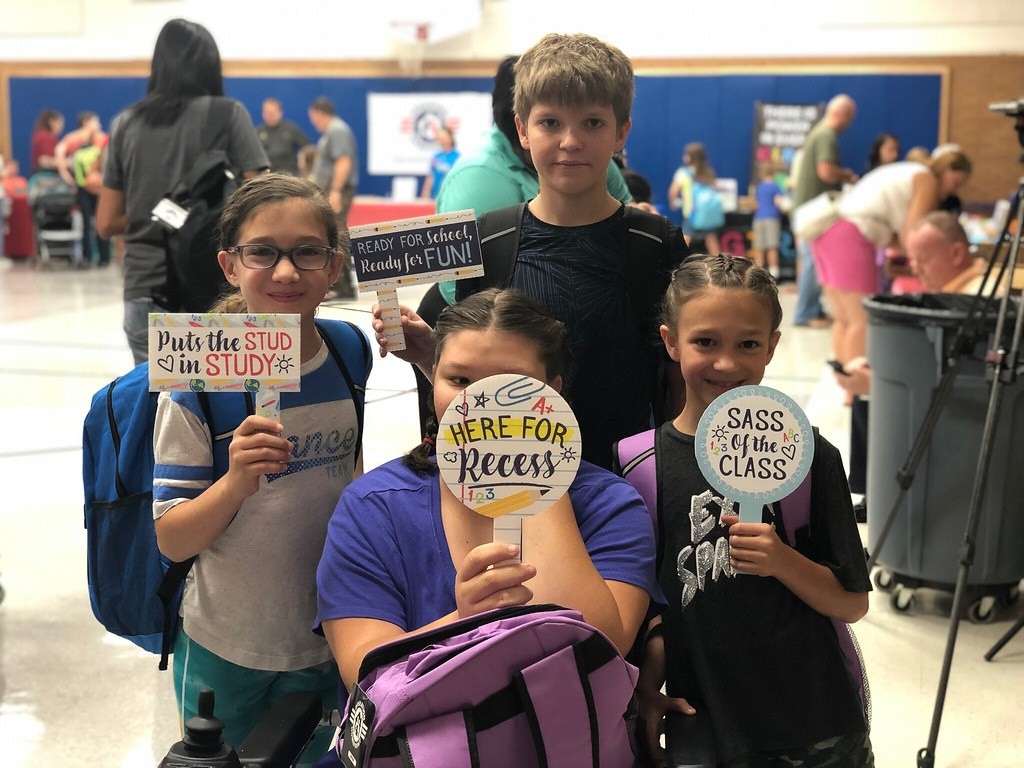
[152,173,375,767]
[667,95,1024,525]
[611,255,875,767]
[374,34,686,472]
[96,18,273,368]
[0,96,358,304]
[312,286,657,768]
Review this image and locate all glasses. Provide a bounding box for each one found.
[226,244,336,271]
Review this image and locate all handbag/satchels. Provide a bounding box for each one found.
[790,191,841,240]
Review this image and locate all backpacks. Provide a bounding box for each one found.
[330,602,642,768]
[682,166,727,232]
[152,95,243,312]
[81,318,365,670]
[73,132,102,186]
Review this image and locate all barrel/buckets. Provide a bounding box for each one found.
[867,295,1024,621]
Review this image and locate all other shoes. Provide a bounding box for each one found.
[853,495,868,523]
[318,285,358,306]
[808,310,837,330]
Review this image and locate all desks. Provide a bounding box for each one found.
[343,197,435,229]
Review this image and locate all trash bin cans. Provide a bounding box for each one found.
[860,291,1023,625]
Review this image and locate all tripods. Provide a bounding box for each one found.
[866,98,1024,768]
